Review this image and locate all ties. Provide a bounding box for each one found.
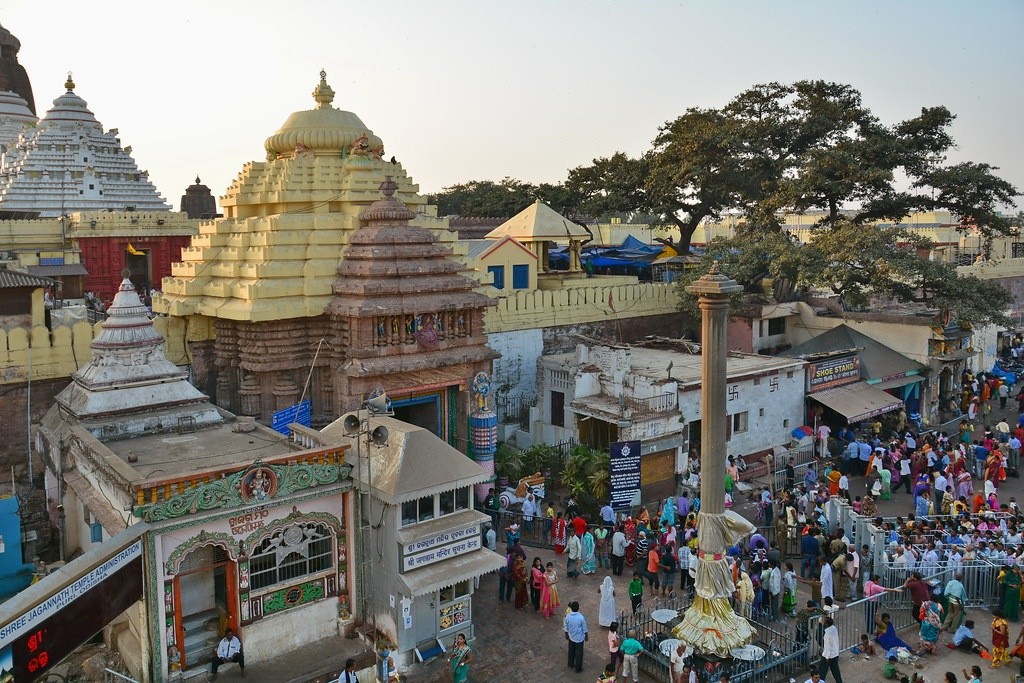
[227,640,230,656]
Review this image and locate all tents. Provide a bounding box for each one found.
[775,322,930,411]
[549,234,743,280]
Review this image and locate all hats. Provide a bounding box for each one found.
[485,522,492,526]
[818,555,826,561]
[813,508,822,513]
[651,542,657,550]
[639,531,645,539]
[510,523,518,530]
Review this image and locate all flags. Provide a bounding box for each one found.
[127,243,146,256]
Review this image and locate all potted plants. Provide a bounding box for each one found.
[494,443,552,485]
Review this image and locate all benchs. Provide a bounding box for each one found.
[413,638,446,663]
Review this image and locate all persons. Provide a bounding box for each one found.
[523,338,1024,683]
[511,554,529,610]
[505,524,520,552]
[447,631,473,683]
[82,286,158,321]
[338,658,360,683]
[483,522,497,574]
[265,147,278,162]
[249,469,268,498]
[209,628,247,682]
[473,375,493,413]
[483,488,495,526]
[494,490,504,524]
[497,551,518,604]
[290,142,308,160]
[44,288,55,331]
[350,137,385,161]
[508,537,527,561]
[482,526,488,546]
[377,311,466,335]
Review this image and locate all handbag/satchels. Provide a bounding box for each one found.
[756,589,762,603]
[929,501,934,515]
[969,424,974,431]
[873,479,882,491]
[791,597,797,605]
[919,608,925,619]
[894,460,901,470]
[843,450,850,460]
[969,484,973,495]
[871,490,881,495]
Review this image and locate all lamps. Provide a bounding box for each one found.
[91,220,96,224]
[159,220,164,225]
[132,219,137,223]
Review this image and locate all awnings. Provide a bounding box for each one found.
[936,350,978,362]
[806,381,904,424]
[397,547,507,599]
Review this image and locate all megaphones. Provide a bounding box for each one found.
[344,414,364,434]
[364,393,387,411]
[369,425,388,445]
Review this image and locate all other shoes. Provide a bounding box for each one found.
[499,598,556,620]
[907,490,914,494]
[892,488,898,494]
